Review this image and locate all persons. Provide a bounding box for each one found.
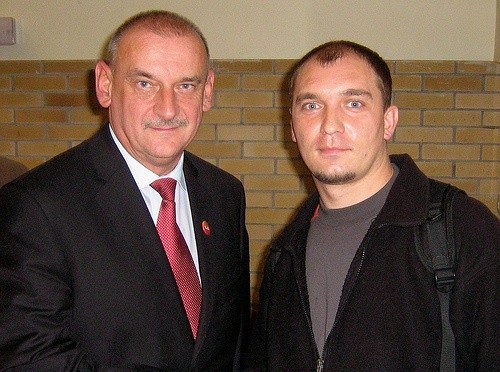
[0,10,254,371]
[243,39,500,372]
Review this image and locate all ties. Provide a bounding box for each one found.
[149,178,203,341]
